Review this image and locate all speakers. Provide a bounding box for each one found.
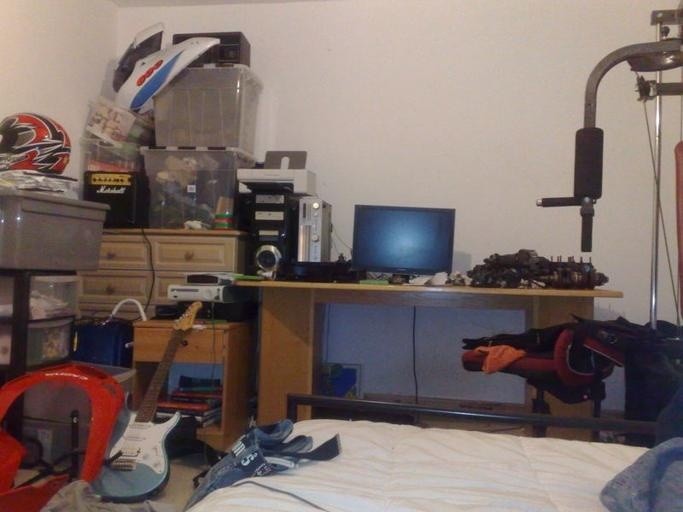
[244,192,298,281]
[82,170,149,228]
[172,31,251,67]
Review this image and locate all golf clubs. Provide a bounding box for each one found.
[256,418,313,469]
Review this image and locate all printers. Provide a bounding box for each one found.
[236,150,317,194]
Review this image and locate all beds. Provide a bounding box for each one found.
[189,417,683,512]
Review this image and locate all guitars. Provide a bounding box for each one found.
[98,301,203,503]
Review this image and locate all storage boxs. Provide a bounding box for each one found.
[0,190,111,274]
[140,146,257,230]
[23,421,128,459]
[23,364,139,425]
[82,138,142,173]
[0,277,83,319]
[84,96,155,159]
[0,317,77,363]
[152,66,267,156]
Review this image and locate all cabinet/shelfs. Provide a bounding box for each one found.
[134,323,250,452]
[229,276,624,440]
[77,230,249,321]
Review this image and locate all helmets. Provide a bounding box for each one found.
[0,112,72,175]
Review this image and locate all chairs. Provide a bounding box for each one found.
[463,326,608,439]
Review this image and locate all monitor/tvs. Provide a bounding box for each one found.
[350,204,456,284]
